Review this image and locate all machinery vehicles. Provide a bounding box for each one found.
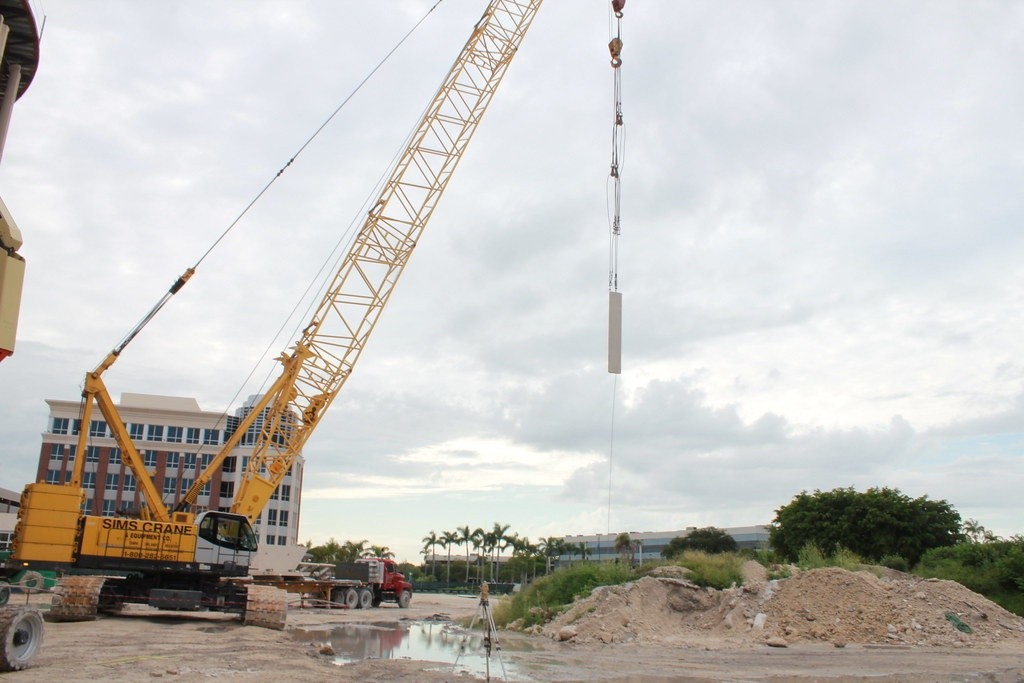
[13,0,626,626]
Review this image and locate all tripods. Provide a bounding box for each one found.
[446,581,508,683]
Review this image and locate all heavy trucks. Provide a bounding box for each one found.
[256,558,412,608]
